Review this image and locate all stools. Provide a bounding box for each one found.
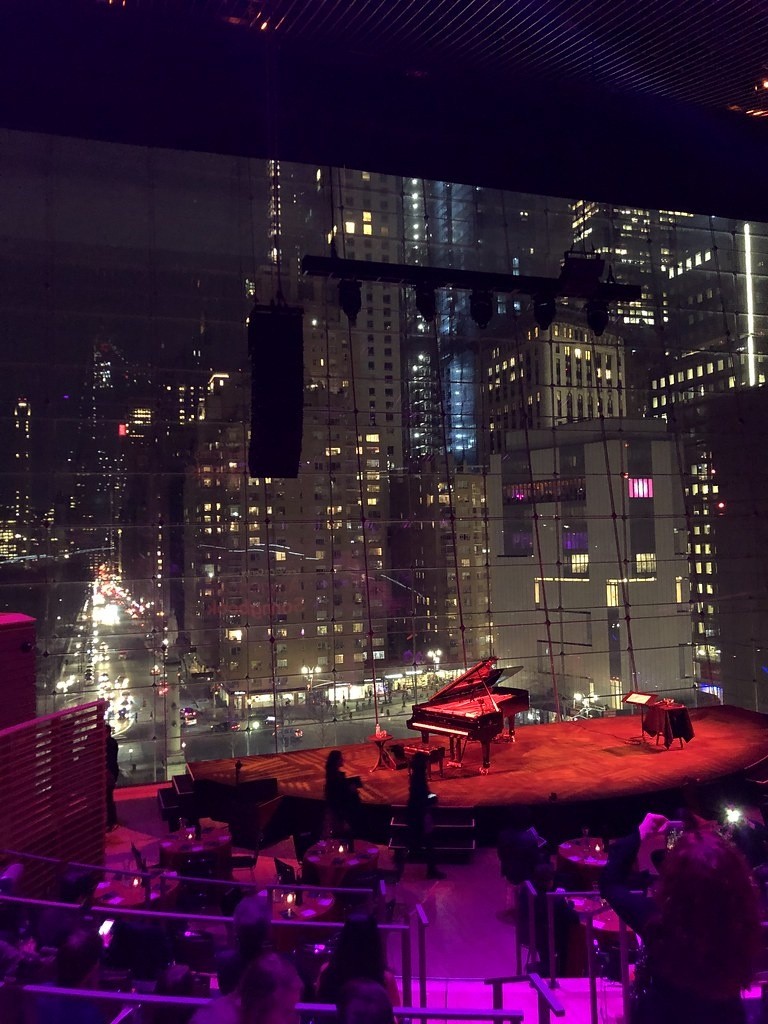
[404,743,445,780]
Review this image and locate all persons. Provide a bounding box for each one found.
[325,750,371,828]
[521,864,570,967]
[189,897,305,1024]
[28,922,110,1023]
[407,752,446,879]
[315,912,394,1024]
[602,813,768,1024]
[105,725,120,830]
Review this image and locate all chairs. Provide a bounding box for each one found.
[497,833,650,974]
[57,833,404,923]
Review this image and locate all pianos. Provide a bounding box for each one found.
[405,656,532,768]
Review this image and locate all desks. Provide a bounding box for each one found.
[559,836,616,891]
[268,882,337,923]
[160,826,232,881]
[367,735,398,772]
[306,836,379,891]
[644,700,695,749]
[95,868,179,913]
[582,908,639,959]
